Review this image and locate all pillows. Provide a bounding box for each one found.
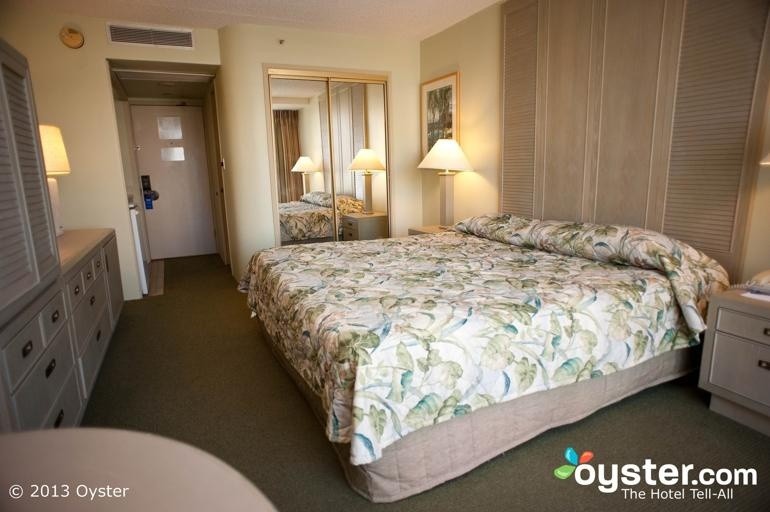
[300,191,364,214]
[446,211,731,343]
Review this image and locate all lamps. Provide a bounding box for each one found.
[758,150,770,167]
[347,148,386,215]
[39,124,73,238]
[417,139,474,230]
[290,155,319,197]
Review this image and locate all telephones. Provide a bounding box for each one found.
[726,269,770,294]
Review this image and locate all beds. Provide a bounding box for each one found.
[237,230,707,504]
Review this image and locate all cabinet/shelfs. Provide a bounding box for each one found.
[0,37,62,331]
[62,242,115,405]
[0,274,86,434]
[100,229,124,334]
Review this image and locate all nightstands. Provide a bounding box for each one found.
[342,212,388,240]
[408,225,443,236]
[698,290,770,438]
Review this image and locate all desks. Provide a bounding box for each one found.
[0,427,280,512]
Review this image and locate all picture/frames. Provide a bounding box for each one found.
[419,70,461,165]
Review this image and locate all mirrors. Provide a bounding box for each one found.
[262,61,395,247]
